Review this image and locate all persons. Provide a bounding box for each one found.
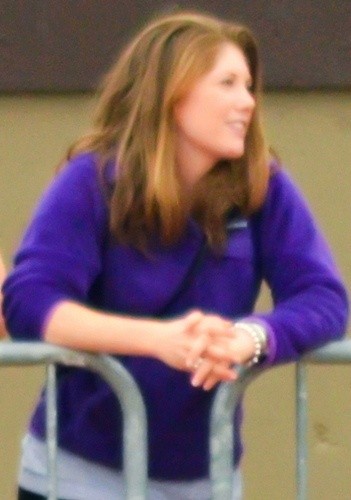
[0,10,350,500]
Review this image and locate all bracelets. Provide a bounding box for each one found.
[234,321,265,368]
